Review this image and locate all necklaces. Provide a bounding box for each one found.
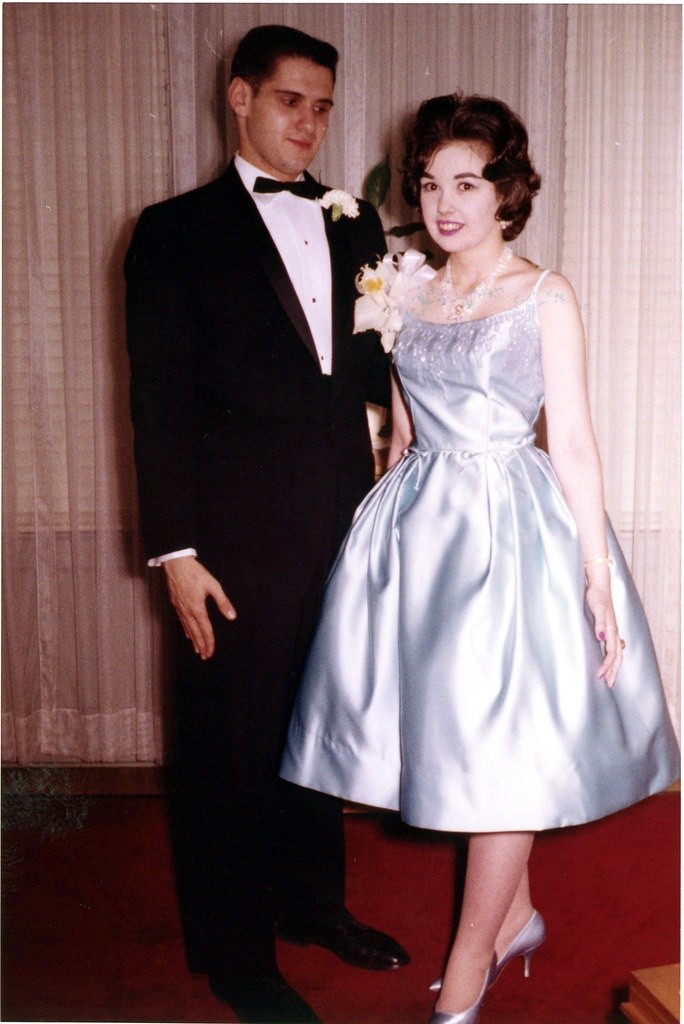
[439,244,512,325]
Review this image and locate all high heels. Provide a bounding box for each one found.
[430,950,498,1024]
[428,908,545,992]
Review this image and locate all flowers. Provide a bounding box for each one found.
[352,248,438,352]
[318,188,360,221]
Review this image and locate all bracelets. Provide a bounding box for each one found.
[582,559,613,568]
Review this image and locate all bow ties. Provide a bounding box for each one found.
[253,175,320,200]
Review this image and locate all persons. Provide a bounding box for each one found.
[123,24,413,1024]
[277,92,681,1024]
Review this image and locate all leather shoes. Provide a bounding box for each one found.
[274,904,411,970]
[209,962,323,1024]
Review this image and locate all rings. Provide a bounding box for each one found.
[618,640,626,648]
